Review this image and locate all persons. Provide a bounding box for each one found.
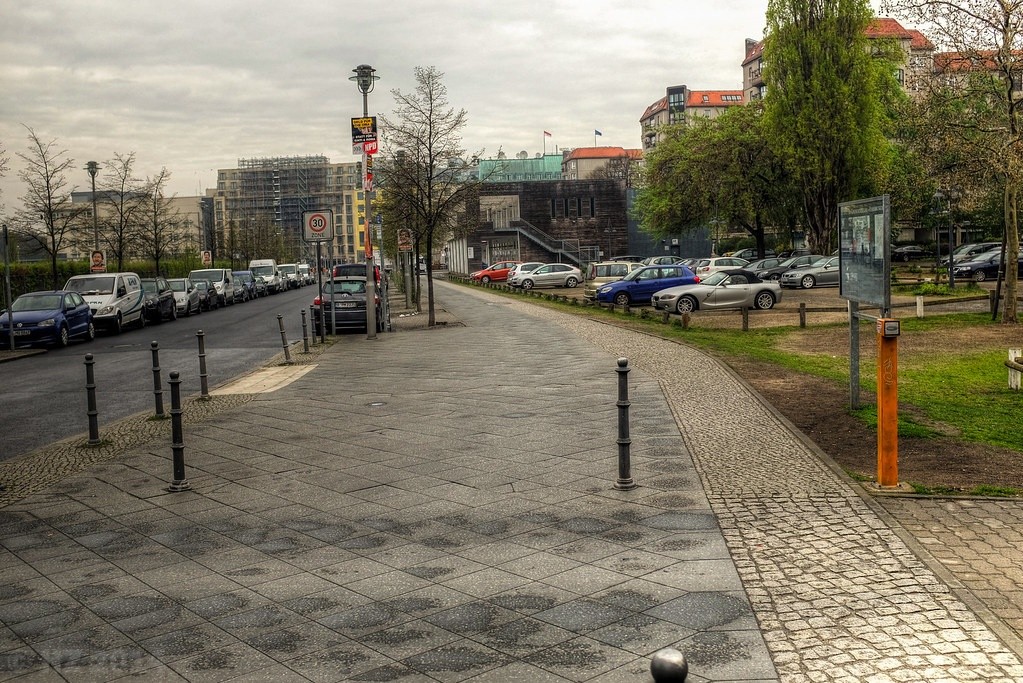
[204,252,210,261]
[399,230,412,246]
[91,251,106,272]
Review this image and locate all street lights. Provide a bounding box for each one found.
[346,63,381,338]
[604,229,617,261]
[708,217,720,256]
[250,218,259,260]
[82,160,103,249]
[198,200,209,250]
[933,188,970,287]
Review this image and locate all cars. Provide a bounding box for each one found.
[254,276,269,296]
[141,277,178,324]
[780,256,839,288]
[506,262,558,288]
[730,247,775,261]
[300,273,306,285]
[233,276,250,303]
[651,269,782,315]
[611,255,702,277]
[311,270,316,283]
[737,257,789,275]
[314,264,388,336]
[947,246,1023,281]
[470,261,527,284]
[512,263,584,290]
[723,252,734,257]
[0,289,95,349]
[595,264,700,306]
[940,243,1023,266]
[278,271,290,291]
[757,255,825,283]
[191,279,220,311]
[891,246,935,262]
[167,278,203,315]
[233,271,259,300]
[696,257,754,279]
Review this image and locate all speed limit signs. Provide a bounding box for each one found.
[309,214,327,233]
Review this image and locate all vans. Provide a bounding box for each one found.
[298,264,313,286]
[278,264,301,289]
[187,268,234,307]
[249,259,280,295]
[415,257,426,274]
[583,261,659,301]
[59,272,147,333]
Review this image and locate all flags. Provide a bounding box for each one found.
[595,130,602,136]
[545,132,551,137]
